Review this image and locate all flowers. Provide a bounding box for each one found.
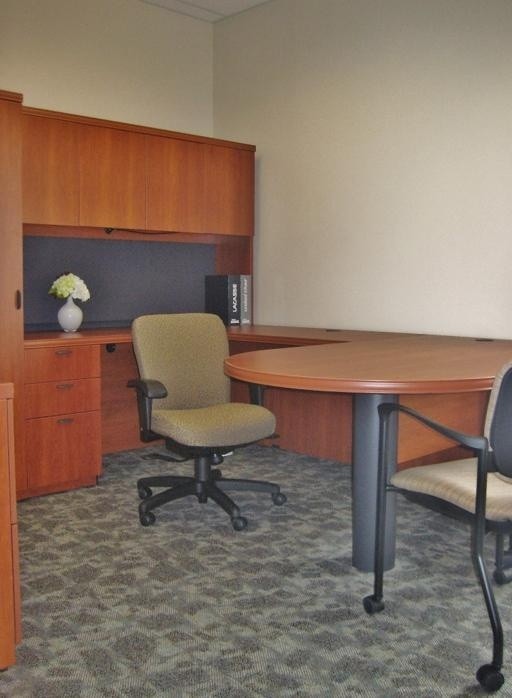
[44,271,91,304]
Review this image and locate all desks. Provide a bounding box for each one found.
[18,321,512,576]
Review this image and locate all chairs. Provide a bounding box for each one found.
[121,309,286,533]
[358,358,512,697]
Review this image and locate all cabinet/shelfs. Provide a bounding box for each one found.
[201,135,257,247]
[20,101,80,241]
[1,375,25,674]
[0,86,29,506]
[78,111,146,244]
[144,123,201,245]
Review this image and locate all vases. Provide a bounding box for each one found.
[56,295,85,334]
